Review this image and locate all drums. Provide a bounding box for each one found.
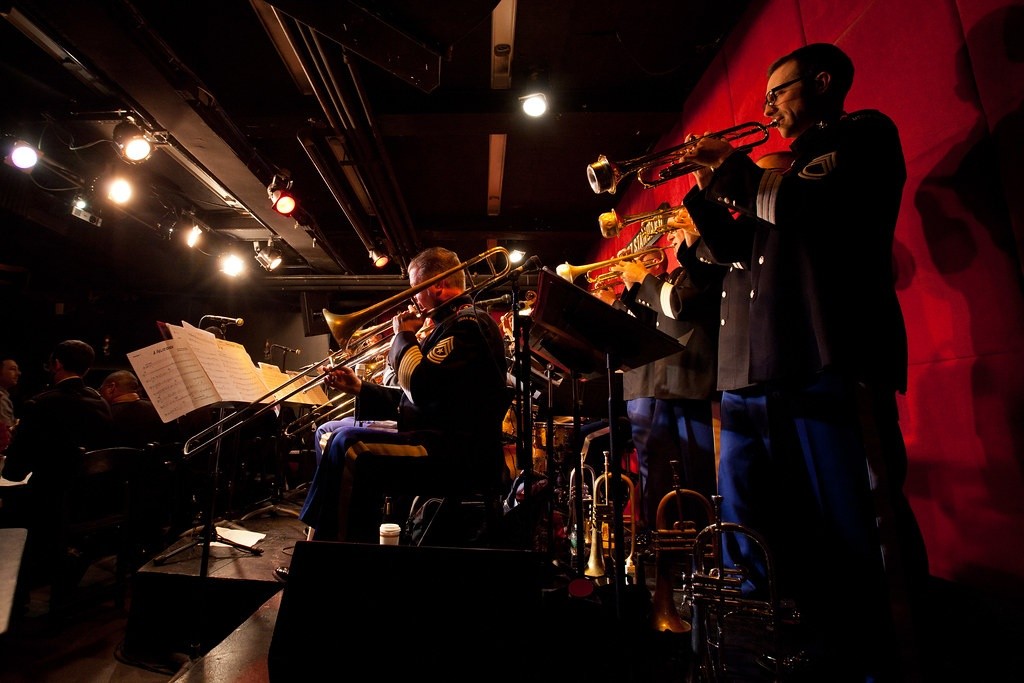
[533,421,577,453]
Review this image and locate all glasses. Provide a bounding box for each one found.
[43,359,56,372]
[761,76,818,110]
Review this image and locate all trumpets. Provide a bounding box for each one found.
[596,205,702,239]
[564,449,814,683]
[553,243,678,295]
[585,119,782,196]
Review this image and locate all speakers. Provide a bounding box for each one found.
[301,291,332,337]
[268,541,534,683]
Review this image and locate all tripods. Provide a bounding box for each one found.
[151,322,333,566]
[534,362,588,587]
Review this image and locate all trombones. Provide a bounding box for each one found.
[181,244,514,460]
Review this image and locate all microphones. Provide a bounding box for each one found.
[484,255,543,290]
[475,294,511,308]
[274,344,301,355]
[204,314,244,326]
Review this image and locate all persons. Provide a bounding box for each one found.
[0,326,163,622]
[272,245,520,586]
[592,148,809,622]
[682,42,949,683]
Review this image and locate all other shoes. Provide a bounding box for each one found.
[273,567,291,583]
